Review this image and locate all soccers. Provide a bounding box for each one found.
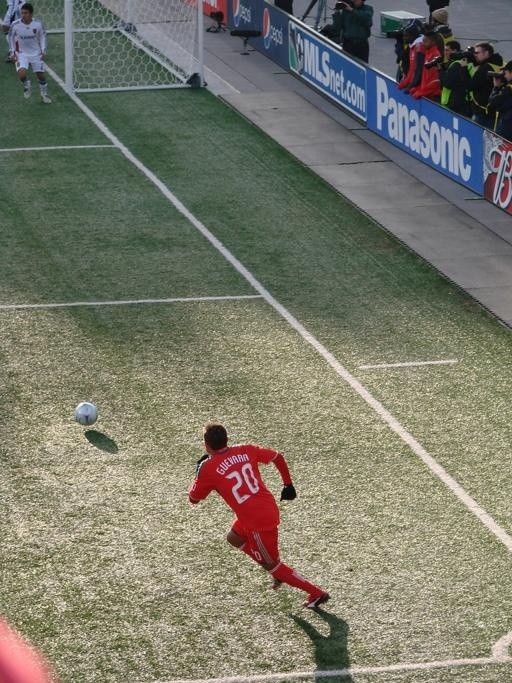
[75,402,96,425]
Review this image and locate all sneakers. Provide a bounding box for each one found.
[22,88,33,100]
[303,590,329,609]
[5,52,17,65]
[40,94,52,105]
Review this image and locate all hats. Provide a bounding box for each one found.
[430,6,450,25]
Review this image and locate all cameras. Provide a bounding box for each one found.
[449,46,476,63]
[424,55,443,68]
[386,30,404,40]
[488,68,509,87]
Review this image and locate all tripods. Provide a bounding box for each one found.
[299,0,328,31]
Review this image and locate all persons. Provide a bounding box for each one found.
[274,0,511,143]
[9,2,55,104]
[188,423,330,610]
[0,0,27,62]
